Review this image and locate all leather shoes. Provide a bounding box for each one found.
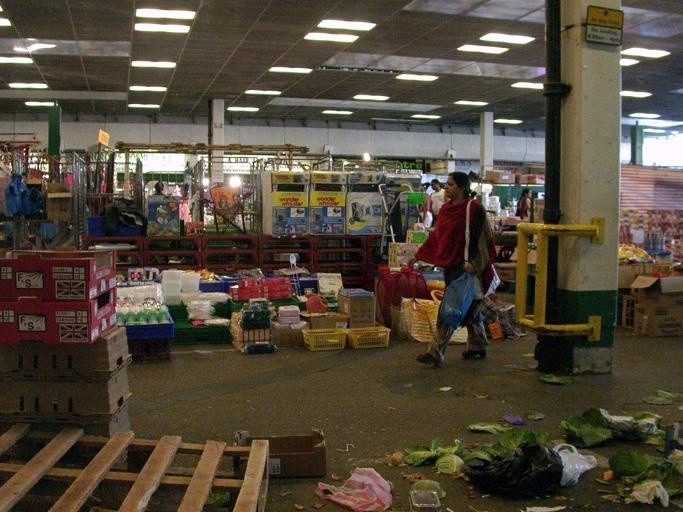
[461,349,486,360]
[416,353,443,367]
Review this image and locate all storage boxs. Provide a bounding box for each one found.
[493,246,537,286]
[631,273,683,337]
[300,295,375,330]
[0,250,133,437]
[234,429,327,480]
[487,170,517,185]
[162,270,199,306]
[45,191,73,222]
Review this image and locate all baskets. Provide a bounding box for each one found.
[301,328,346,352]
[347,326,391,350]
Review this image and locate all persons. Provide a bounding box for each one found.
[430,179,452,226]
[409,171,496,363]
[154,182,165,195]
[417,187,433,228]
[518,189,533,217]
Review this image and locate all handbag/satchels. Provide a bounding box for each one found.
[472,263,501,301]
[424,290,468,345]
